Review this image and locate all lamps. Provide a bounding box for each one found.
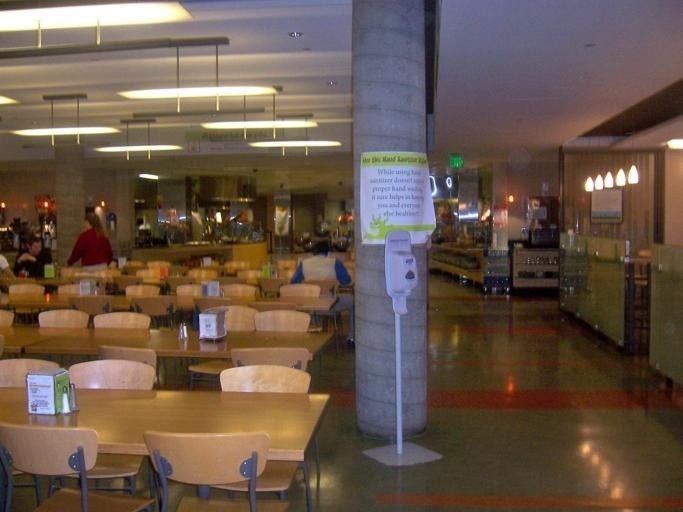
[1,1,197,50]
[249,112,341,158]
[92,115,184,159]
[2,423,157,510]
[10,92,123,148]
[201,94,320,140]
[116,43,280,115]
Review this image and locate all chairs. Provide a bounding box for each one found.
[0,256,354,389]
[210,363,312,492]
[3,358,59,474]
[144,428,292,510]
[64,360,158,477]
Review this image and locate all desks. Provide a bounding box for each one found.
[1,388,331,511]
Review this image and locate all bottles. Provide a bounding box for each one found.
[67,382,79,412]
[177,322,187,340]
[59,385,70,414]
[522,253,556,264]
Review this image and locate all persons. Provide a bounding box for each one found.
[65,212,113,269]
[13,237,53,294]
[290,238,354,349]
[0,253,16,306]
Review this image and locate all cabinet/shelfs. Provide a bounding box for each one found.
[431,242,488,284]
[512,247,559,287]
